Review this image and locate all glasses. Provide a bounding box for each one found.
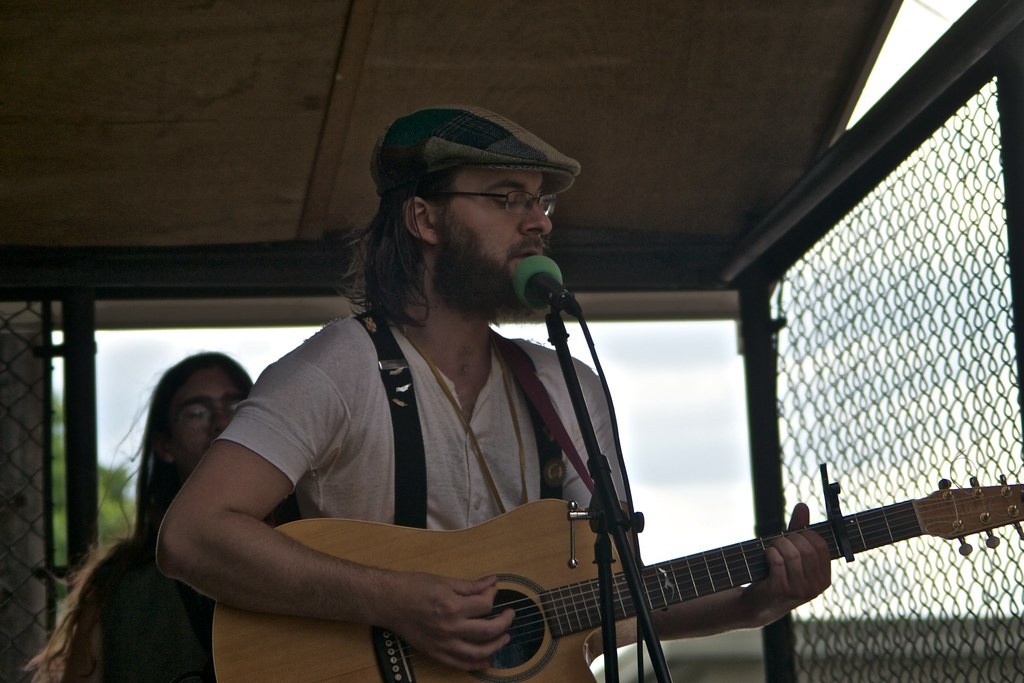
[432,188,558,218]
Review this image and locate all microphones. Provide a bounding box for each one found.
[512,255,582,317]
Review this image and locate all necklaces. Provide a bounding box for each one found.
[397,328,529,515]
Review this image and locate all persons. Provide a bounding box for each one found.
[156,105,831,683]
[30,351,253,683]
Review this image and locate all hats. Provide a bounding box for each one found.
[367,102,582,198]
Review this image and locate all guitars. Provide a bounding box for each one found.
[209,461,1024,682]
